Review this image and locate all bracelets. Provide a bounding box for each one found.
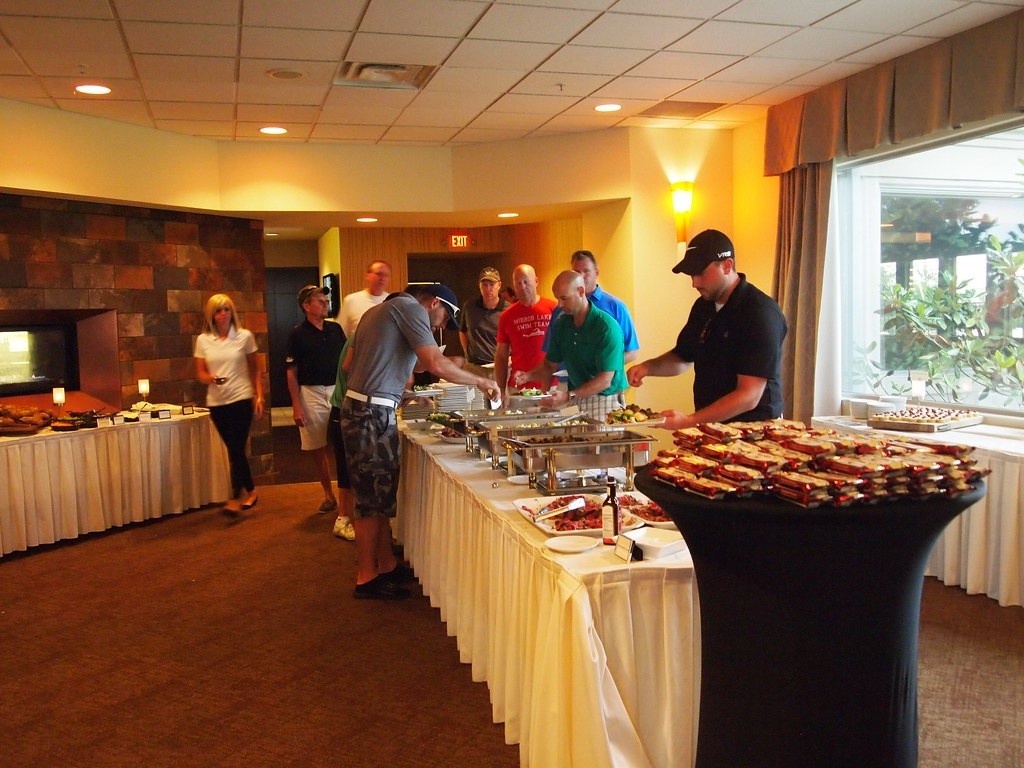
[258,398,265,403]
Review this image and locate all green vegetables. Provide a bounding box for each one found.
[412,384,434,392]
[428,411,452,424]
[520,388,546,396]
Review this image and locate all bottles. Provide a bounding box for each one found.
[602,476,621,546]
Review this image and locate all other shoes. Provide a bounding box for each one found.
[241,493,258,507]
[223,499,240,512]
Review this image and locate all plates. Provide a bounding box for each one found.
[506,475,529,484]
[553,370,570,378]
[605,413,666,427]
[435,430,466,443]
[508,394,553,401]
[512,492,687,559]
[403,345,511,413]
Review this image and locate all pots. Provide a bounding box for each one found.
[48,421,80,431]
[66,406,106,417]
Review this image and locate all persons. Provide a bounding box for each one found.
[627,229,788,431]
[327,284,501,599]
[193,294,264,517]
[285,285,347,513]
[458,267,519,365]
[538,270,630,417]
[335,261,393,334]
[542,250,640,383]
[493,264,558,392]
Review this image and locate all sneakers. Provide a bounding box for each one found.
[319,500,337,513]
[333,520,355,540]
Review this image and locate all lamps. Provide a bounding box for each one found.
[669,181,694,255]
[137,378,150,402]
[52,388,65,418]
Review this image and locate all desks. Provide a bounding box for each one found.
[633,461,987,768]
[811,415,1024,608]
[0,409,233,557]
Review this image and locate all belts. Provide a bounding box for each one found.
[346,390,398,409]
[468,359,492,365]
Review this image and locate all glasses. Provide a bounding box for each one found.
[306,300,330,306]
[211,375,227,385]
[436,296,461,318]
[699,313,717,344]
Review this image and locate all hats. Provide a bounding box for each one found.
[672,229,736,275]
[478,267,501,282]
[297,285,330,304]
[422,284,462,330]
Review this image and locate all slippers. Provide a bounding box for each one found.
[389,566,416,582]
[353,581,410,599]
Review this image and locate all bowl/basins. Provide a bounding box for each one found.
[213,377,228,384]
[404,419,428,429]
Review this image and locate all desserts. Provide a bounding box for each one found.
[873,408,980,423]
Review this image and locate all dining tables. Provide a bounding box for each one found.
[386,416,704,768]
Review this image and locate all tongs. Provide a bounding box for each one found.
[533,498,585,522]
